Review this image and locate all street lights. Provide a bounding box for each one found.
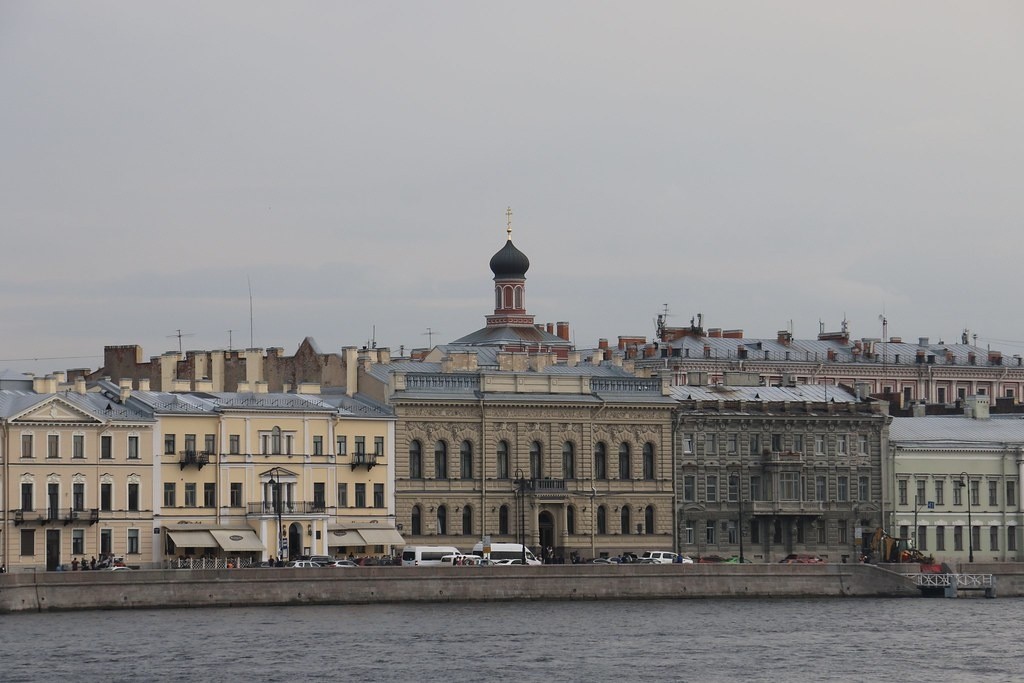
[959,471,974,563]
[912,494,927,552]
[267,467,284,564]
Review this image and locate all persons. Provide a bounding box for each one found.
[71,554,123,571]
[859,554,868,563]
[455,555,474,565]
[365,553,402,566]
[542,545,555,564]
[673,553,683,563]
[227,559,238,569]
[349,551,354,558]
[570,551,586,564]
[617,554,632,564]
[0,564,6,573]
[903,552,908,562]
[269,554,274,566]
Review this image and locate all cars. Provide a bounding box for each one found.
[328,560,360,567]
[473,558,533,566]
[310,555,334,566]
[106,566,132,571]
[244,562,270,567]
[720,557,753,563]
[699,555,725,563]
[779,553,831,564]
[587,552,662,565]
[284,560,321,568]
[354,555,402,566]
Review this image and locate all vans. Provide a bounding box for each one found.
[440,554,483,566]
[400,546,463,568]
[473,541,542,566]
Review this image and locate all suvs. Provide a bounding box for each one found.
[641,551,694,564]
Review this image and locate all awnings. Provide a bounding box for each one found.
[326,523,405,547]
[164,523,267,552]
